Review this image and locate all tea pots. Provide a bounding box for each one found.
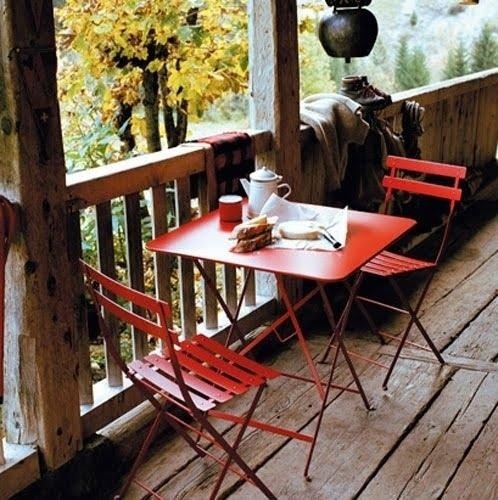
[240,166,291,219]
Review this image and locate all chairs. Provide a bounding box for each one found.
[317,153,473,392]
[78,257,286,500]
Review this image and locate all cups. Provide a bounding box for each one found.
[219,195,243,222]
